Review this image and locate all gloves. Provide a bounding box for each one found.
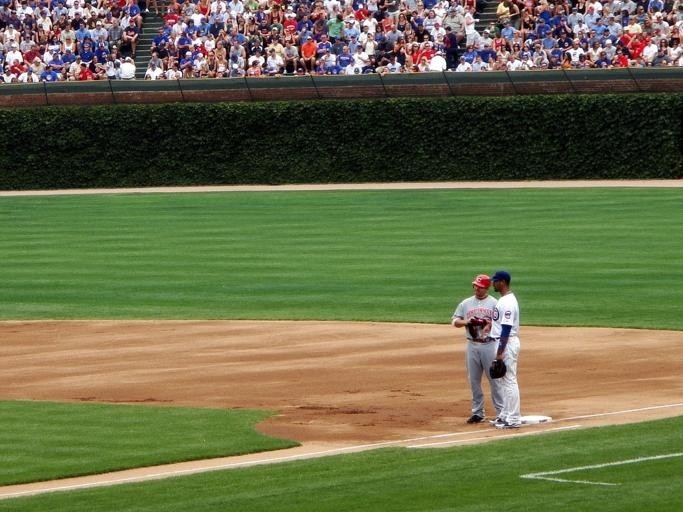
[467,315,485,329]
[491,360,508,379]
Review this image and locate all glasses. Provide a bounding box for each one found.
[0,1,682,70]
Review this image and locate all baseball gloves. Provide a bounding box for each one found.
[490,359,506,378]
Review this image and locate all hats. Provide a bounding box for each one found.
[469,274,492,290]
[489,270,510,283]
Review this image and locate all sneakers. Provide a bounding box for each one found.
[488,416,516,430]
[465,414,482,425]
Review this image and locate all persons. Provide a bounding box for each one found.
[0,1,683,88]
[450,273,503,424]
[488,271,521,430]
